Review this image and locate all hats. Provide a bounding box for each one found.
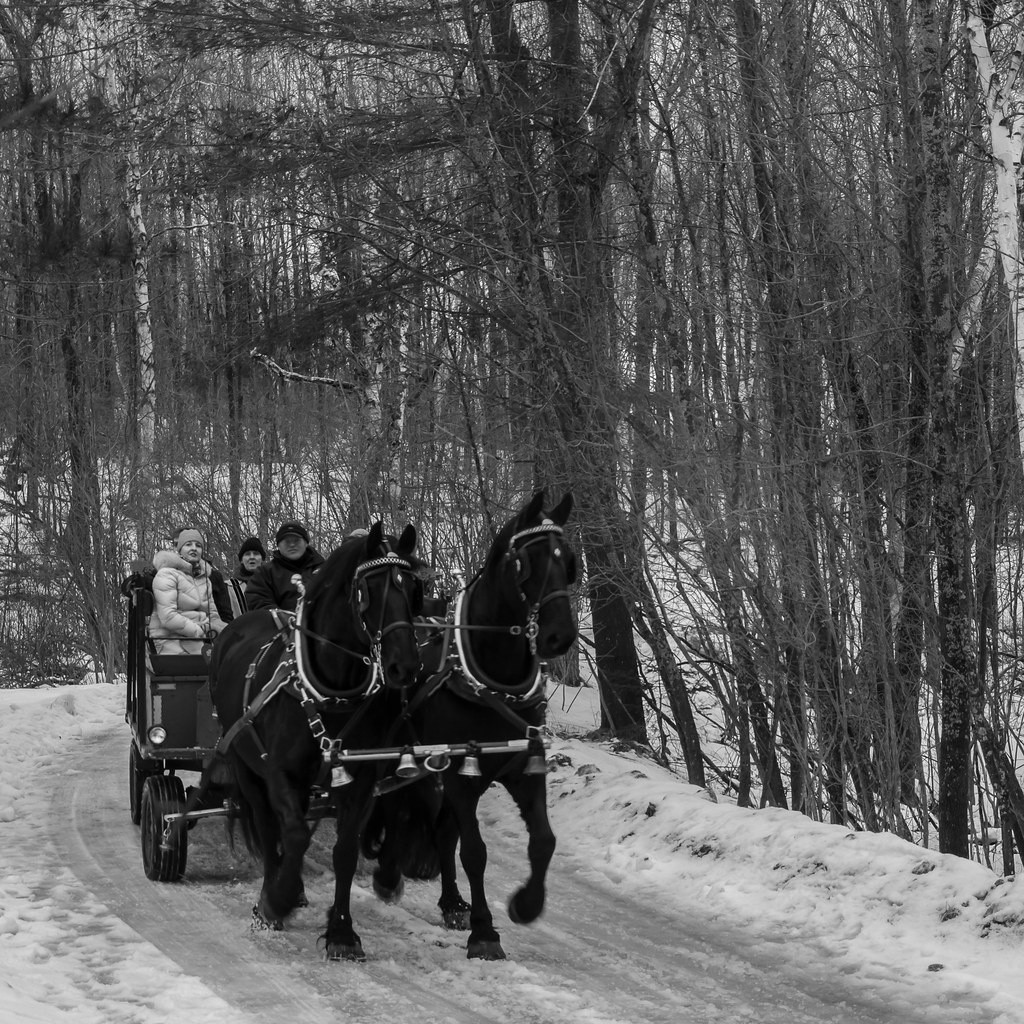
[238,537,266,562]
[275,523,309,546]
[177,529,204,554]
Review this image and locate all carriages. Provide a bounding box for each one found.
[119,485,587,964]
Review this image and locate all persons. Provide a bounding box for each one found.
[222,537,270,623]
[244,523,327,613]
[121,527,235,624]
[150,527,230,657]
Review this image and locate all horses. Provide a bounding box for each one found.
[205,488,585,965]
